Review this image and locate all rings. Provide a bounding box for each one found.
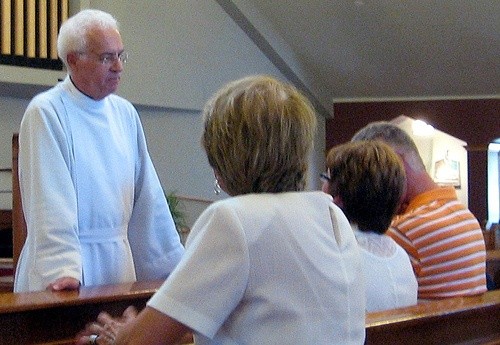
[89,335,97,345]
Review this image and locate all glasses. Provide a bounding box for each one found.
[78,51,129,67]
[320,170,331,184]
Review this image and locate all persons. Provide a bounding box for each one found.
[14,9,186,292]
[76,76,367,345]
[350,121,487,300]
[320,141,417,312]
